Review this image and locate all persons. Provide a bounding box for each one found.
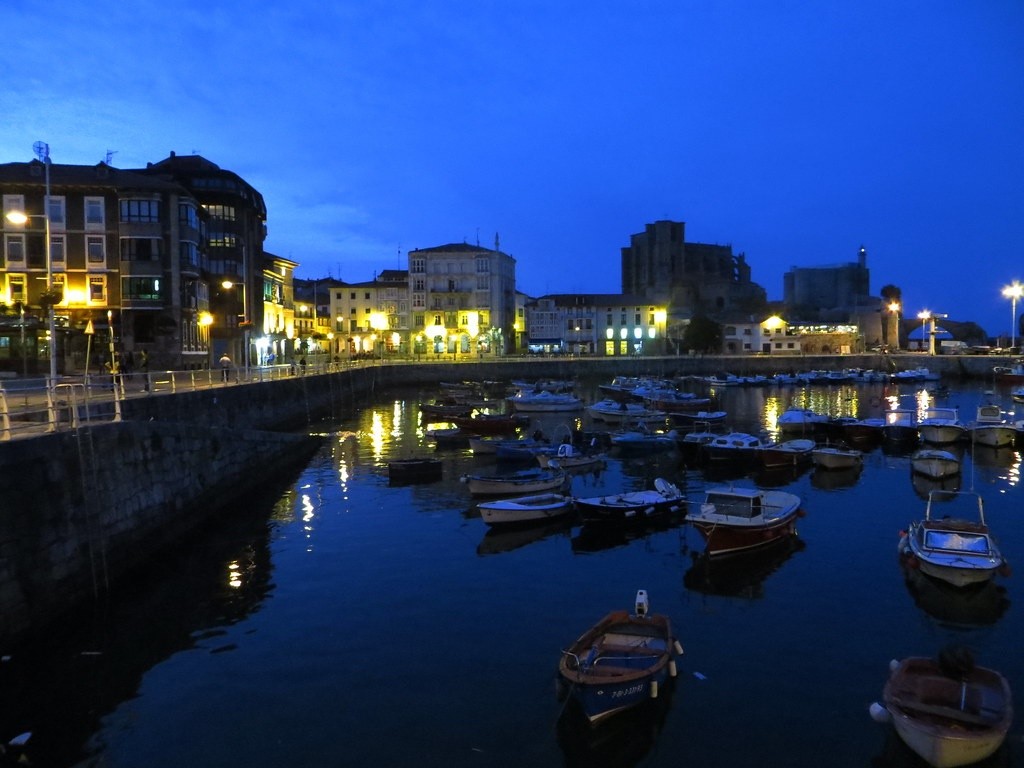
[334,356,340,372]
[289,356,306,375]
[97,347,149,393]
[219,352,232,381]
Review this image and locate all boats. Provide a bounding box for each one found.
[388,456,444,485]
[477,490,577,530]
[683,482,806,560]
[564,477,689,538]
[883,656,1014,768]
[897,488,1008,590]
[418,363,1024,498]
[558,589,684,728]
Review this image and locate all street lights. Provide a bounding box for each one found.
[6,209,57,403]
[1002,282,1024,349]
[917,309,931,342]
[221,281,250,381]
